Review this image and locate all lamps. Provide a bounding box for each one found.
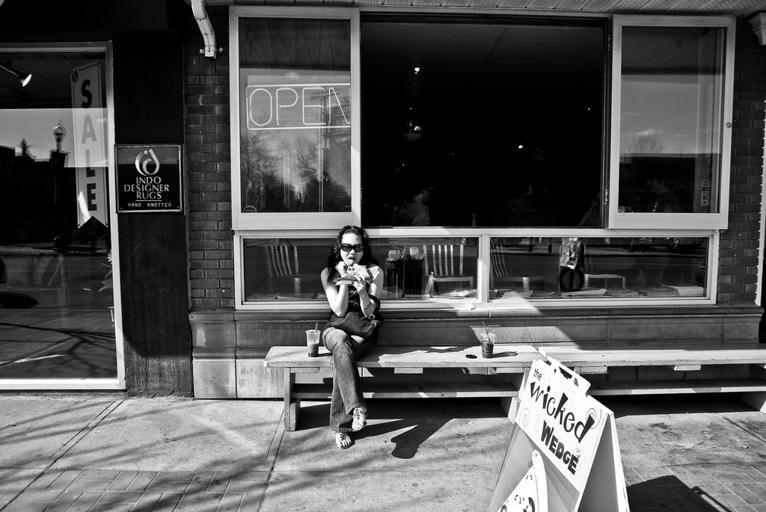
[0,63,32,87]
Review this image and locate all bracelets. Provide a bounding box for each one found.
[360,299,371,309]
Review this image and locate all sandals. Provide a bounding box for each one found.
[351,406,367,432]
[335,432,352,450]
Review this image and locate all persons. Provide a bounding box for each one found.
[321,225,384,449]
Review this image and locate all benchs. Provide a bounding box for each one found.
[264,345,549,432]
[538,343,766,413]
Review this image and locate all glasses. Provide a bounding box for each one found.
[340,243,364,252]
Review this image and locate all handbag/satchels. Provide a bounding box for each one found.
[330,294,383,340]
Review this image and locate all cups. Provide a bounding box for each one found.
[305,329,322,357]
[480,332,497,358]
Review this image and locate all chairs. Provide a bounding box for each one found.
[490,237,548,290]
[423,238,474,297]
[558,240,627,292]
[265,239,320,293]
